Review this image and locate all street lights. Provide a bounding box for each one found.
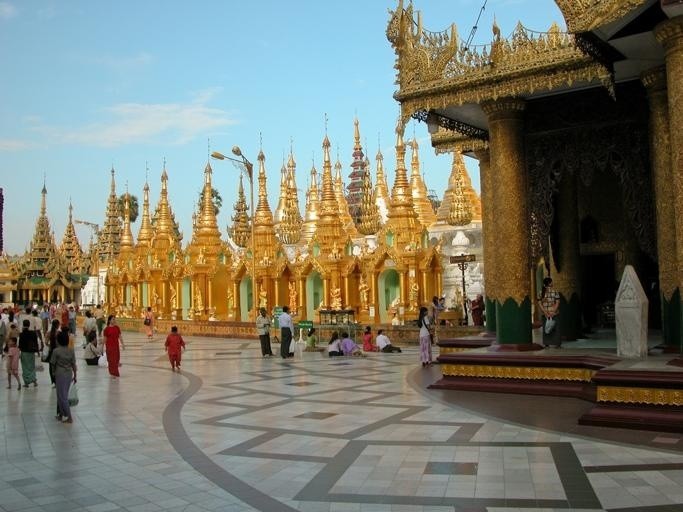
[210,147,255,321]
[75,219,101,311]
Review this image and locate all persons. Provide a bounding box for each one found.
[392,313,400,325]
[304,328,325,352]
[418,296,445,368]
[0,299,124,423]
[468,293,485,327]
[328,326,401,357]
[144,307,155,339]
[278,306,295,358]
[537,278,560,349]
[164,326,185,369]
[256,307,276,356]
